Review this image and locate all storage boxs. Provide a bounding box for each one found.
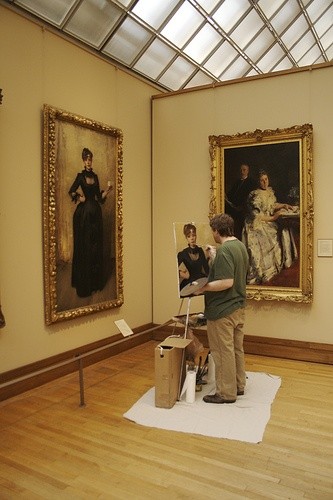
[154,337,193,410]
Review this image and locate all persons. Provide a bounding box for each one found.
[192,214,249,403]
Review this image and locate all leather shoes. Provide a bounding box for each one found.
[237,391,244,395]
[203,393,236,403]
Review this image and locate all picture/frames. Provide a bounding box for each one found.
[41,103,125,327]
[208,121,315,305]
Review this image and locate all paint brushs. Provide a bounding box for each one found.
[196,350,211,385]
[190,243,216,248]
